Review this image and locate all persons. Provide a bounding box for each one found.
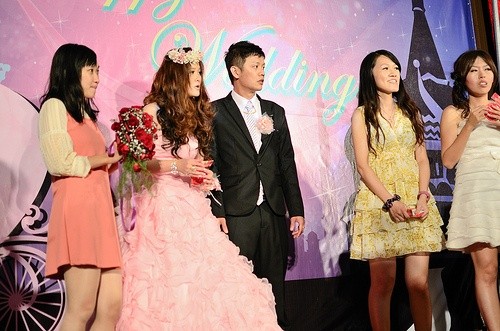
[440,50,500,331]
[39,43,125,331]
[352,50,446,331]
[114,47,285,331]
[211,40,305,331]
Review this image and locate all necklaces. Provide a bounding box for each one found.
[384,110,393,121]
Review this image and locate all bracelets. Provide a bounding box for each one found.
[384,194,400,209]
[417,191,430,201]
[171,161,176,170]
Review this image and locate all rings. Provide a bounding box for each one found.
[302,227,305,229]
[193,167,195,170]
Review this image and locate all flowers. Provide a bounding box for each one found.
[256,112,276,135]
[168,49,202,64]
[108,105,158,216]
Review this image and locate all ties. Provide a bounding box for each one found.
[245,102,264,206]
